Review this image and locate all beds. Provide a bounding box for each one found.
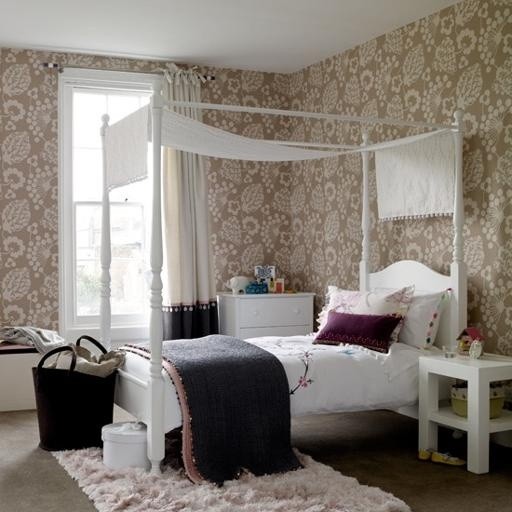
[96,82,464,477]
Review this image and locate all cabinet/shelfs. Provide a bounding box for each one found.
[0,327,58,415]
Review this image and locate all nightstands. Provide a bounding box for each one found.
[216,291,315,339]
[418,351,512,475]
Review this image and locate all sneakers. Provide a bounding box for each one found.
[431,451,465,466]
[418,447,437,461]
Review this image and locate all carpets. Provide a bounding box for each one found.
[51,438,411,512]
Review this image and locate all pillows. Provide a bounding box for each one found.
[312,281,449,355]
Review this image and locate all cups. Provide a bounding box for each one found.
[442,344,458,361]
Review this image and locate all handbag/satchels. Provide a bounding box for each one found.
[32,336,120,450]
